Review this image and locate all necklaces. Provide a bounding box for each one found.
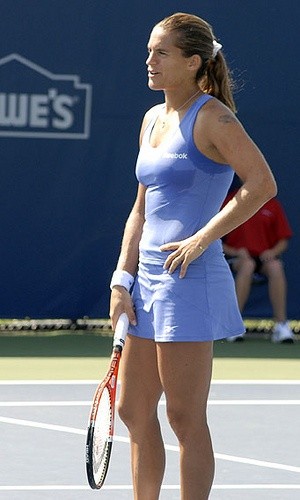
[162,90,201,128]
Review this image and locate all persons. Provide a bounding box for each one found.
[219,190,296,344]
[108,13,277,500]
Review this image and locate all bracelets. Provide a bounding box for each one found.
[110,270,134,292]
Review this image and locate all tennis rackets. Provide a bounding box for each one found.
[83,303,136,491]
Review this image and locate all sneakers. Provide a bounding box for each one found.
[272,320,294,344]
[227,333,244,342]
